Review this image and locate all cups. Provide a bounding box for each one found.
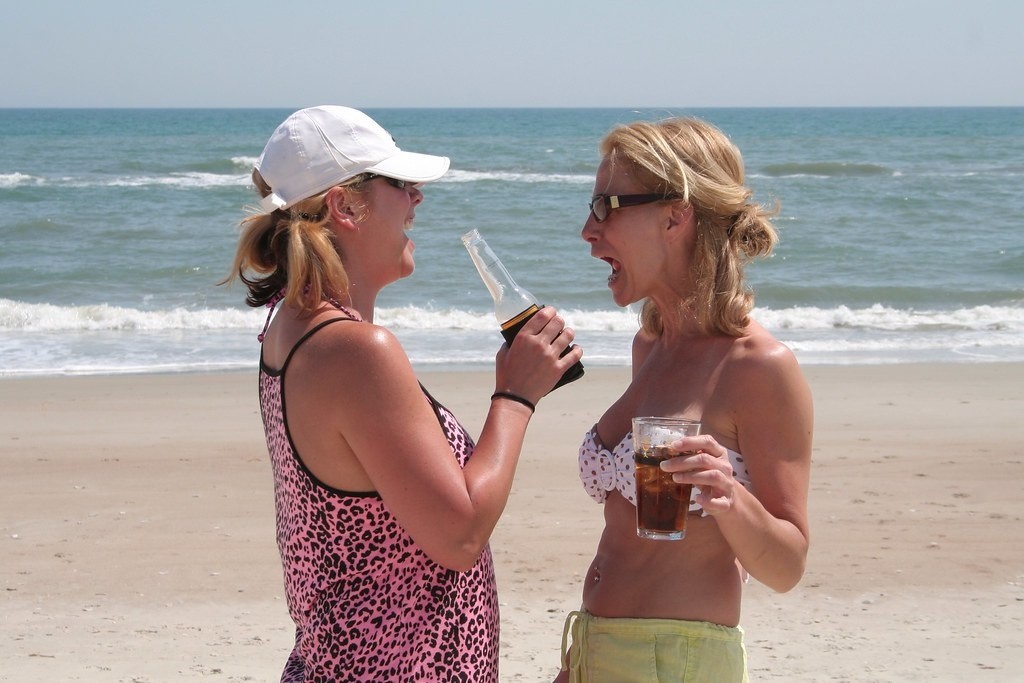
[631,417,701,540]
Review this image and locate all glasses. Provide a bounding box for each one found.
[360,173,404,188]
[588,193,676,223]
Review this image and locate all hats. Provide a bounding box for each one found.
[254,105,451,214]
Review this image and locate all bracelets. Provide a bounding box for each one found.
[490,390,535,412]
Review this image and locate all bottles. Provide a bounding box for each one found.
[462,229,584,398]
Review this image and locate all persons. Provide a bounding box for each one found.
[215,106,582,682]
[553,115,814,682]
